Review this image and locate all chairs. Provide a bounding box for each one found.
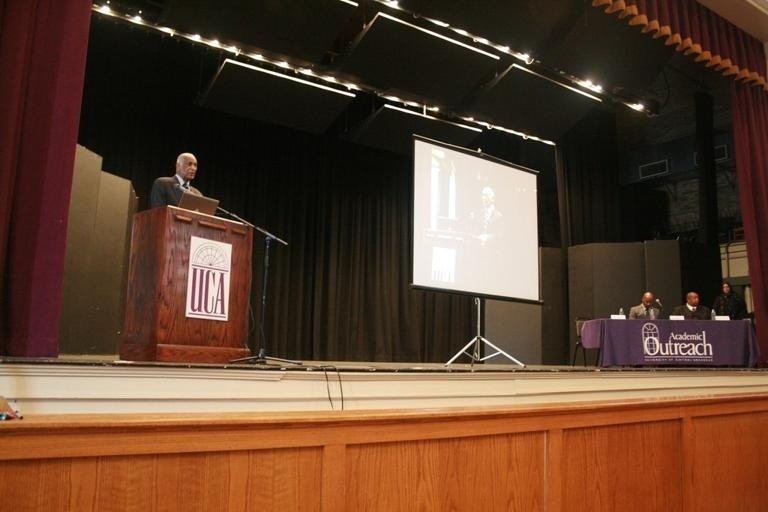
[572,316,599,367]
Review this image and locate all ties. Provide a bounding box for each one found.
[692,308,696,313]
[182,183,188,190]
[645,307,650,320]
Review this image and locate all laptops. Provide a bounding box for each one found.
[178,191,221,216]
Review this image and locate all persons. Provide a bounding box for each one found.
[469,186,505,244]
[150,152,197,208]
[671,291,711,320]
[629,291,660,320]
[712,282,748,320]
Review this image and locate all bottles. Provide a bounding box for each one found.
[619,308,624,315]
[711,309,716,320]
[650,309,655,320]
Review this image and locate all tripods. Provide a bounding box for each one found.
[444,296,527,369]
[217,206,302,366]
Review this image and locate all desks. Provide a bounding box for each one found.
[584,318,752,368]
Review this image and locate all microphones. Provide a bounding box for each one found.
[174,182,187,192]
[656,298,662,308]
